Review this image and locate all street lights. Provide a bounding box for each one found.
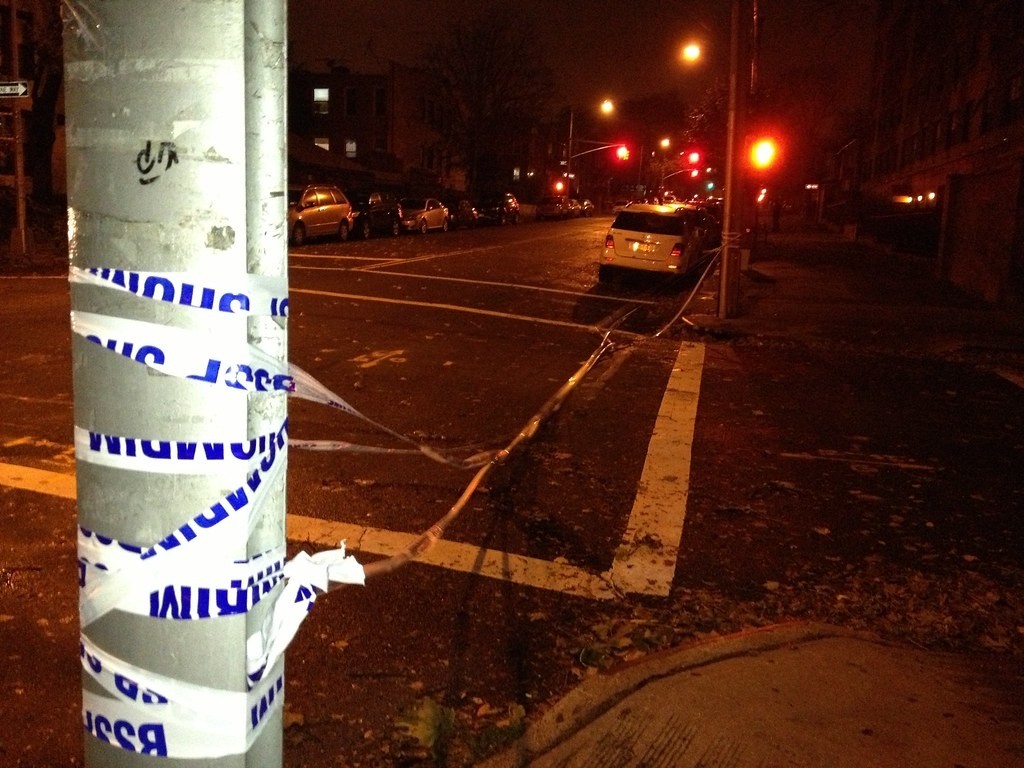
[565,143,629,201]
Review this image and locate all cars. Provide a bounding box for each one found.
[535,196,595,221]
[673,195,725,249]
[399,197,450,236]
[439,196,479,231]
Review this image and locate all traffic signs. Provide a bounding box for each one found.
[0,81,29,98]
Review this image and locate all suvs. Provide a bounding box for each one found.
[597,199,701,294]
[287,181,355,247]
[344,189,404,241]
[471,191,521,226]
